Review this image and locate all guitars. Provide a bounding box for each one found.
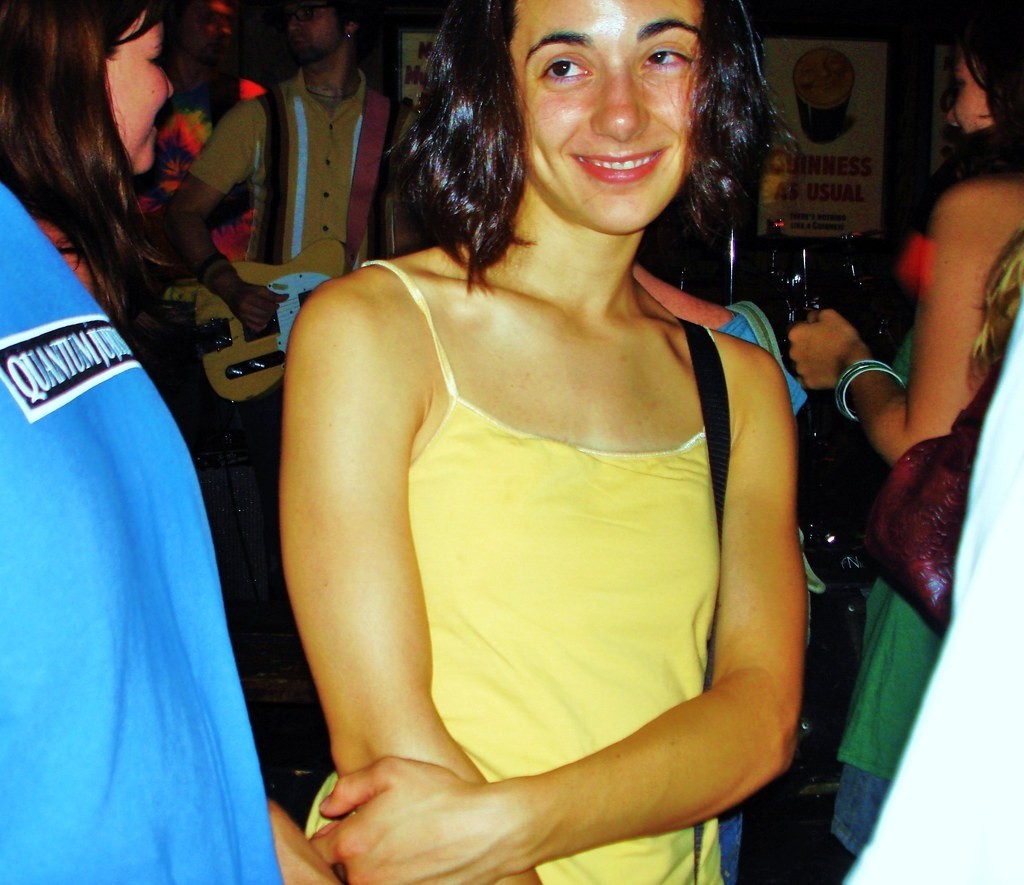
[194,238,347,402]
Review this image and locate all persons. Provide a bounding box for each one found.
[126,0,434,704]
[0,183,342,885]
[613,4,1017,885]
[1,0,177,339]
[268,0,800,882]
